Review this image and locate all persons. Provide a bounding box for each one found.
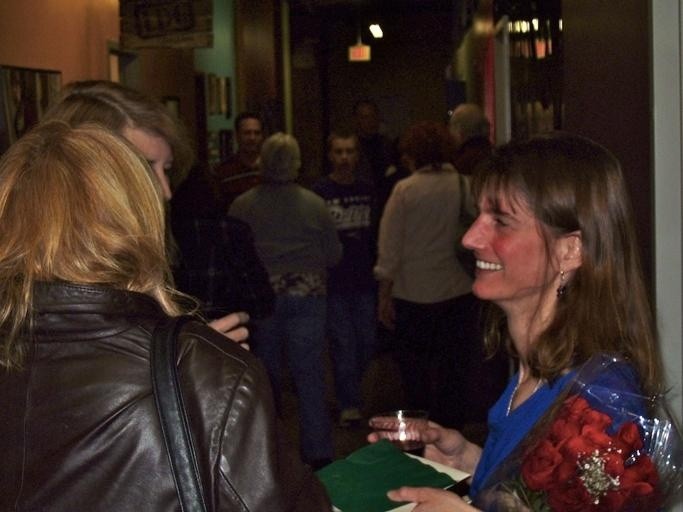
[369,133,665,512]
[2,119,334,512]
[34,79,519,472]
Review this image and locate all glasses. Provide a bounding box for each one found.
[448,110,457,118]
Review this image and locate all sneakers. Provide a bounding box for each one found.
[312,460,332,471]
[339,408,363,426]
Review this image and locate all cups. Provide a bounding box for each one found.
[371,410,428,458]
